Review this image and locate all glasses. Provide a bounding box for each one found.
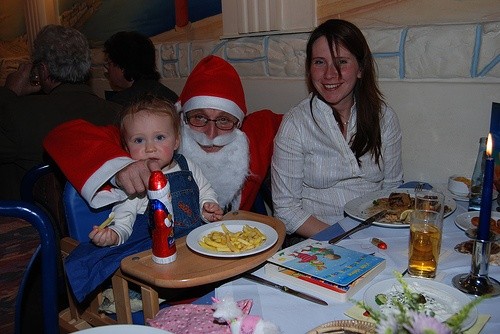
[185,113,238,130]
[103,61,117,73]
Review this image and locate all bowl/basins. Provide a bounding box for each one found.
[447,178,470,196]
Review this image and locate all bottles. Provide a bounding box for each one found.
[468,137,487,212]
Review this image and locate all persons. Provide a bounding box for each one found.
[271,19,404,249]
[0,24,119,215]
[63,97,222,312]
[101,29,179,104]
[44,55,284,216]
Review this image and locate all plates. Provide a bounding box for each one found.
[452,188,498,201]
[186,219,278,257]
[363,278,477,332]
[70,325,171,334]
[344,188,456,227]
[454,211,500,231]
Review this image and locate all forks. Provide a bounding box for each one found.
[414,182,423,192]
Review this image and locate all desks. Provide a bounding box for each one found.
[193,183,500,334]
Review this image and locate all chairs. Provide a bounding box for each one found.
[59,182,286,332]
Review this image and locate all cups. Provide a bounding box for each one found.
[415,190,445,260]
[29,65,42,86]
[408,209,443,279]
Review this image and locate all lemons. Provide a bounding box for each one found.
[401,210,418,221]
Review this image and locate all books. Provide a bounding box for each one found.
[242,237,386,307]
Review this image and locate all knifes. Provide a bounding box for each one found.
[329,209,388,244]
[242,272,329,306]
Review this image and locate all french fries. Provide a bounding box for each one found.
[199,224,266,252]
[98,215,114,231]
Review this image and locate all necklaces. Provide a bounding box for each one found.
[336,121,348,125]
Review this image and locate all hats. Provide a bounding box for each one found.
[175,55,247,128]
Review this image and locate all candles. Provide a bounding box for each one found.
[477,133,494,240]
